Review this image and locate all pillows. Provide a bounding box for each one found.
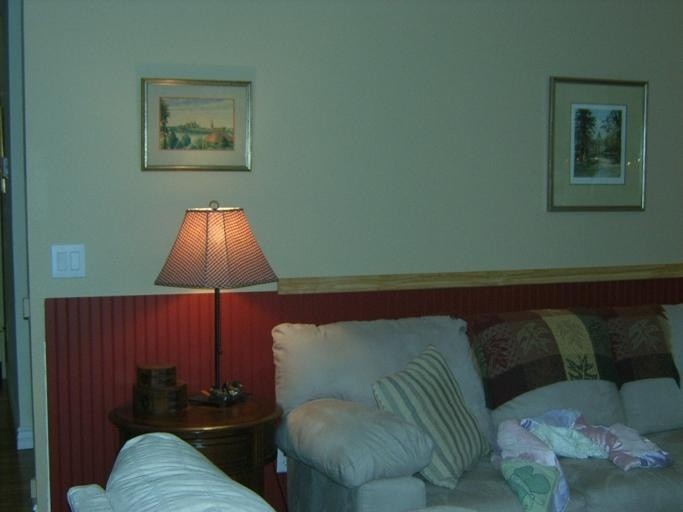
[106,432,276,512]
[464,306,627,450]
[376,343,493,489]
[270,313,493,435]
[278,395,438,490]
[579,303,682,438]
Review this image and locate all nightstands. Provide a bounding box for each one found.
[113,400,282,491]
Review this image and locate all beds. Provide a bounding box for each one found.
[287,423,682,512]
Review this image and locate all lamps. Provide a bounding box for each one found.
[154,200,279,406]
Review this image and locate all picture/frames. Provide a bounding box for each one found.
[547,76,649,213]
[141,78,253,172]
